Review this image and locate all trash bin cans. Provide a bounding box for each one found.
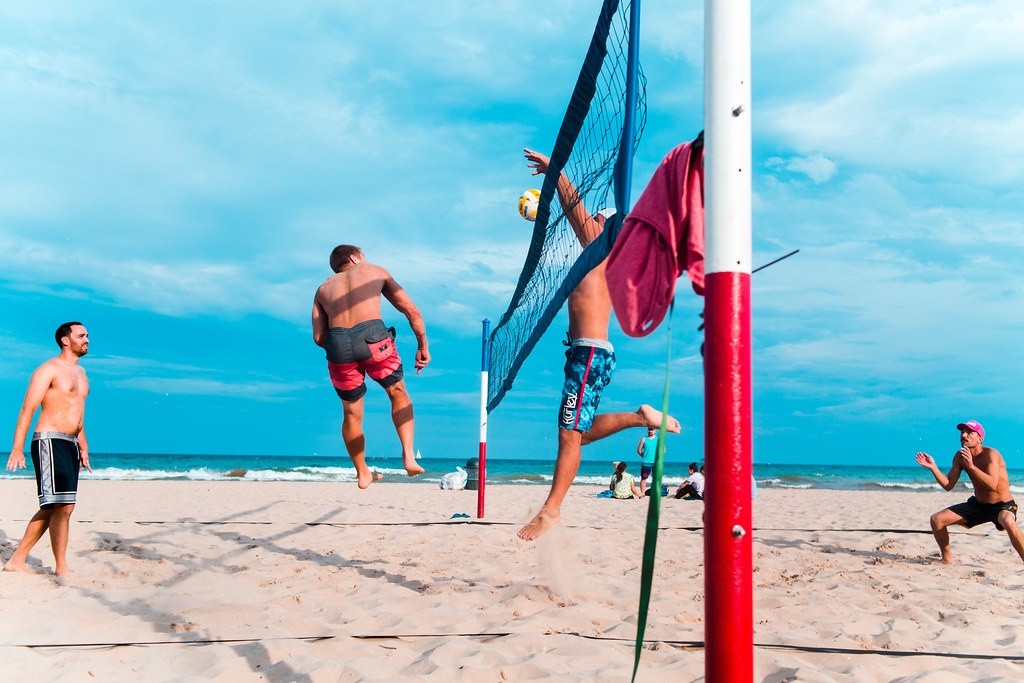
[463,457,479,490]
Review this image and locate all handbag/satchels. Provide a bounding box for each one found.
[441,466,469,490]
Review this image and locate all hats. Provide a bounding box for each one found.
[957,420,984,439]
[598,208,617,219]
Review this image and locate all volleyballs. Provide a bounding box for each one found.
[518,188,542,222]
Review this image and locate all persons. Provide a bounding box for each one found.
[636,424,667,498]
[3,322,94,582]
[311,245,432,490]
[609,460,623,491]
[674,462,705,500]
[747,472,758,501]
[517,146,681,540]
[914,419,1023,568]
[610,461,644,499]
[698,465,708,499]
